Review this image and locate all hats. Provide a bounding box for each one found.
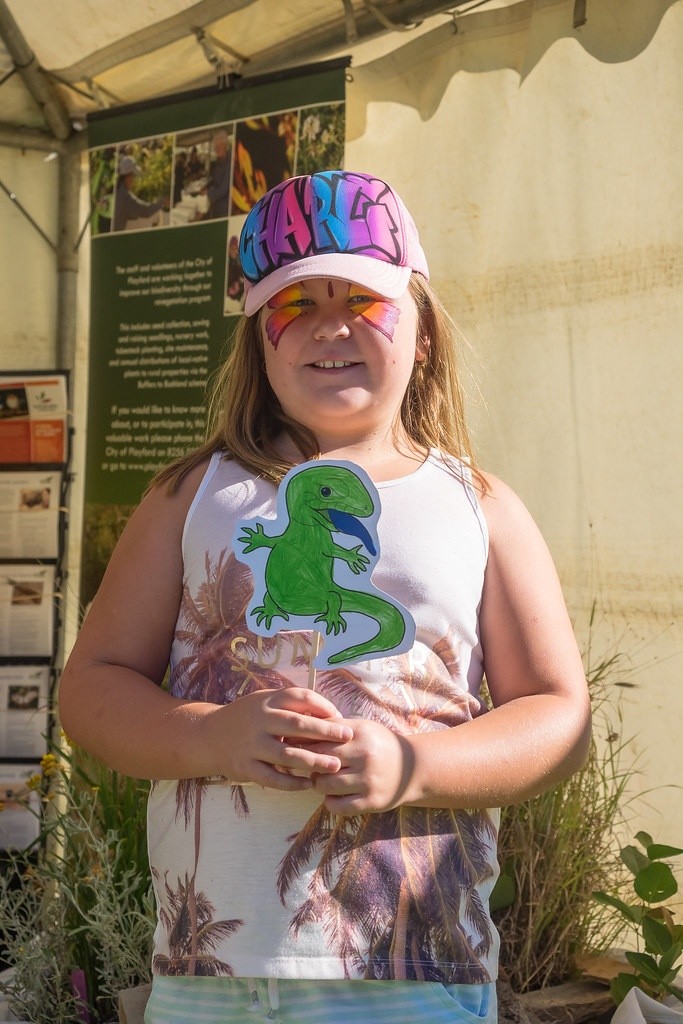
[238,169,429,317]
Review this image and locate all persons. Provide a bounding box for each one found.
[229,236,243,300]
[173,129,232,219]
[115,156,170,230]
[55,170,592,1024]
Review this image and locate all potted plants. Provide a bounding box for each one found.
[491,597,683,1024]
